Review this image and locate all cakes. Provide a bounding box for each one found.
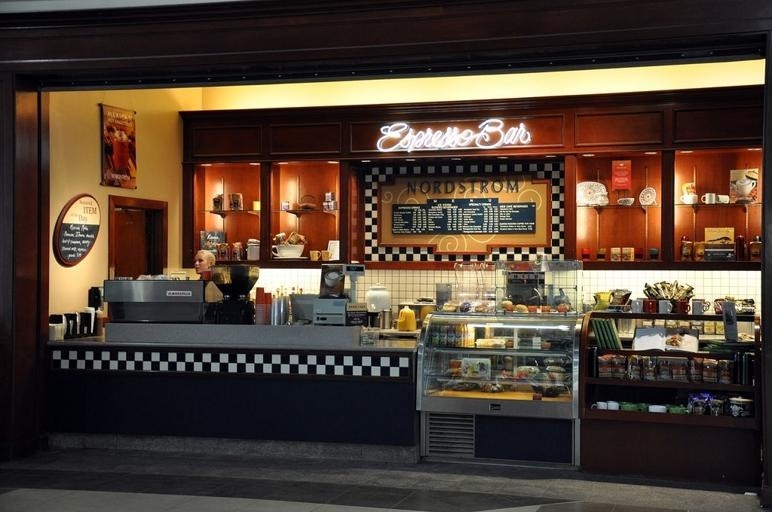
[681,240,713,262]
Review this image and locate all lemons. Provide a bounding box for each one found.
[733,178,758,196]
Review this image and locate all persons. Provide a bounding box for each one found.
[193,249,216,281]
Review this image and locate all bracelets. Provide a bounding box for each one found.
[617,198,635,206]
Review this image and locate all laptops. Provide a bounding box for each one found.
[475,303,490,313]
[443,300,459,312]
[500,301,513,311]
[516,304,527,313]
[558,303,567,312]
[528,305,537,312]
[459,302,471,312]
[540,305,550,312]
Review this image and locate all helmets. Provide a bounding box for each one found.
[576,181,607,207]
[638,186,656,205]
[273,257,309,262]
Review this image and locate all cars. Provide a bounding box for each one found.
[680,193,730,205]
[309,249,332,261]
[214,243,260,261]
[253,285,286,325]
[581,246,636,262]
[631,298,724,314]
[271,244,304,257]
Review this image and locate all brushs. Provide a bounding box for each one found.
[207,261,260,324]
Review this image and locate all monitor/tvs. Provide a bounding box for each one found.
[699,338,755,386]
[632,326,699,353]
[591,317,623,350]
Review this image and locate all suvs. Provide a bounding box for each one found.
[398,306,416,331]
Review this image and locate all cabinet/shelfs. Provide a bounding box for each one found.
[212,192,244,211]
[578,310,764,489]
[681,235,762,261]
[564,142,771,271]
[180,153,364,271]
[365,282,393,312]
[416,308,584,471]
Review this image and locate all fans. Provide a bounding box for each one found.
[391,198,536,234]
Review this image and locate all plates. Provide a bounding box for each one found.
[564,142,771,271]
[180,153,364,271]
